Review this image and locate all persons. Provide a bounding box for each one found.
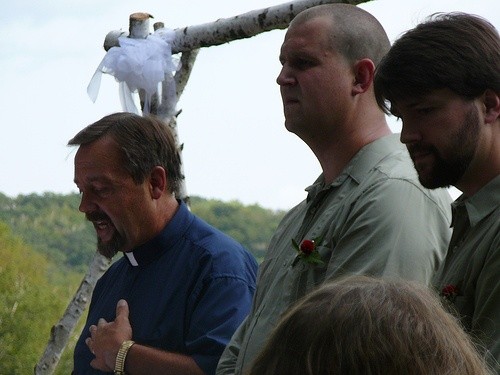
[372,10,500,375]
[68,112,258,375]
[250,272,495,375]
[216,6,453,375]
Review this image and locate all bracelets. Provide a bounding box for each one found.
[113,340,135,375]
